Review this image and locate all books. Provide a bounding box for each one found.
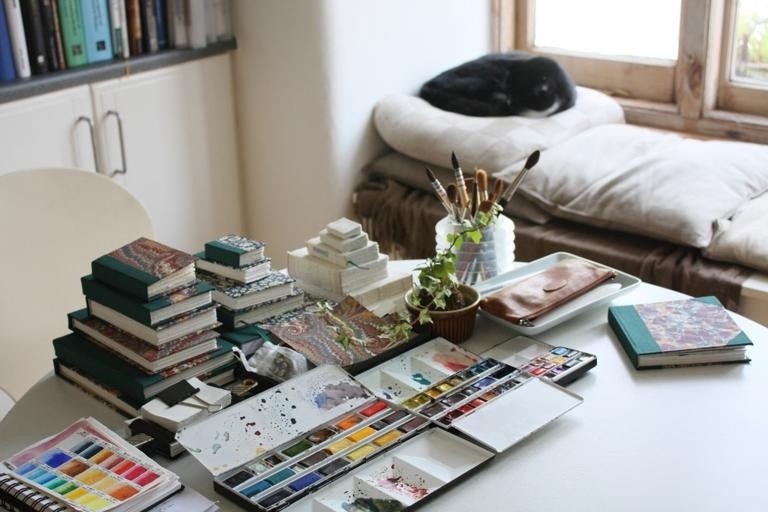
[44,214,423,456]
[602,292,757,375]
[0,0,230,83]
[0,414,187,512]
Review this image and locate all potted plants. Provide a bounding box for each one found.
[321,225,486,353]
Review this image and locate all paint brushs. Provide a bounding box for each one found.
[424,150,540,286]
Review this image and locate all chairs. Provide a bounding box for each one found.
[0,164,157,406]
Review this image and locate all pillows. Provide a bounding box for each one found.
[368,155,550,227]
[369,79,628,184]
[487,113,768,254]
[700,189,768,274]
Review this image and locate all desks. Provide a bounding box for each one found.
[0,249,767,512]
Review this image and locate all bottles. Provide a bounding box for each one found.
[437,214,515,284]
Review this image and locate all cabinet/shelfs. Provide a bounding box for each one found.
[0,43,259,418]
